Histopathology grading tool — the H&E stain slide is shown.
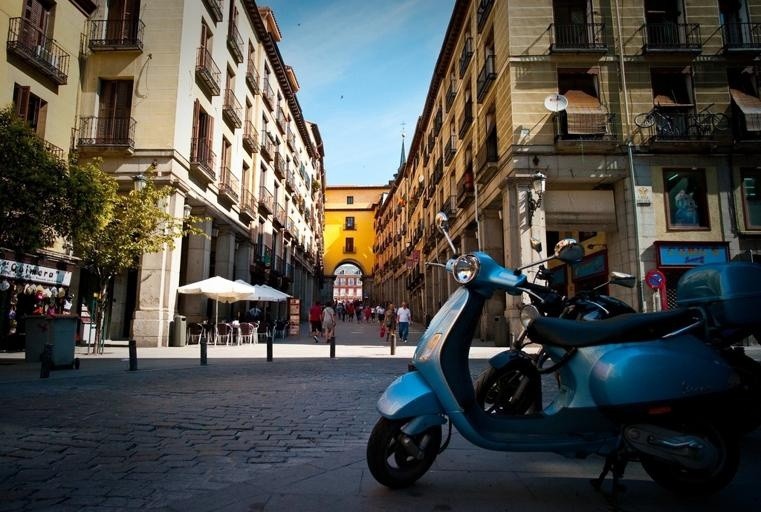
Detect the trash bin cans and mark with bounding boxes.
[24,314,82,371]
[172,315,187,347]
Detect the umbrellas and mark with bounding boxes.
[178,275,293,346]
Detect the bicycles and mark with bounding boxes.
[686,101,730,137]
[633,101,680,136]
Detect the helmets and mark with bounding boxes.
[473,351,542,418]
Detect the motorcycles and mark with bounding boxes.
[473,235,760,435]
[367,211,760,501]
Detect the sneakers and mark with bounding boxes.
[313,336,319,342]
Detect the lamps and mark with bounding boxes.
[526,171,548,227]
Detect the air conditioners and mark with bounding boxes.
[34,45,58,75]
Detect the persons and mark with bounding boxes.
[310,301,412,343]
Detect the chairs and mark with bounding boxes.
[186,320,291,347]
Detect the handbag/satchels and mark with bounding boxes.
[380,327,384,337]
[386,320,393,327]
